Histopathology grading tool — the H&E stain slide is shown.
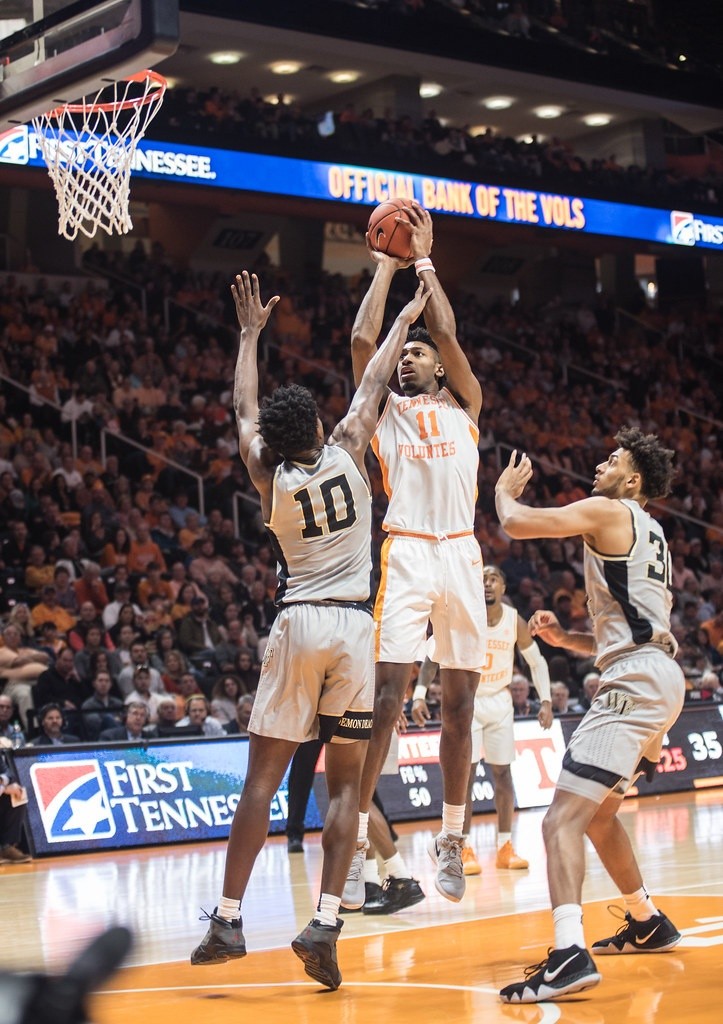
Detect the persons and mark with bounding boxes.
[0,242,723,863]
[412,566,554,875]
[350,0,696,74]
[342,203,487,909]
[191,271,435,991]
[27,86,723,216]
[494,427,686,1004]
[287,712,425,916]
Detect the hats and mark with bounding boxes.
[0,927,133,1024]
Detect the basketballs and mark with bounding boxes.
[368,198,428,260]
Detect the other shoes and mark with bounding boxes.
[1,842,24,861]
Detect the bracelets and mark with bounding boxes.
[415,257,436,276]
[413,685,428,701]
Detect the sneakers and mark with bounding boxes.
[292,918,343,990]
[363,879,383,903]
[287,836,303,853]
[461,848,480,874]
[341,838,370,910]
[191,907,246,967]
[592,904,681,954]
[363,876,425,915]
[500,950,603,1004]
[497,840,531,870]
[428,835,470,902]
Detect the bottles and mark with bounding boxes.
[11,720,22,750]
